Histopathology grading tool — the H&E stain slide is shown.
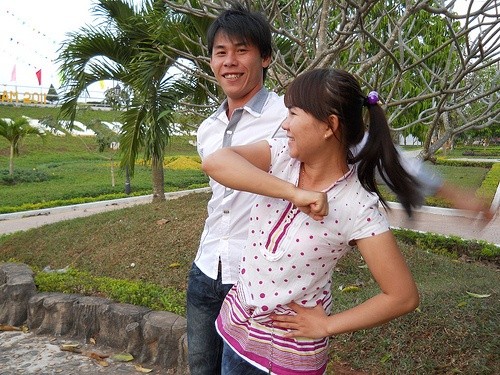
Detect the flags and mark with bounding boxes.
[99,69,105,89]
[10,65,17,82]
[36,70,41,85]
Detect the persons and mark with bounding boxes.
[198,67,428,375]
[186,2,497,375]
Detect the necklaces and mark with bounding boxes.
[302,163,305,190]
